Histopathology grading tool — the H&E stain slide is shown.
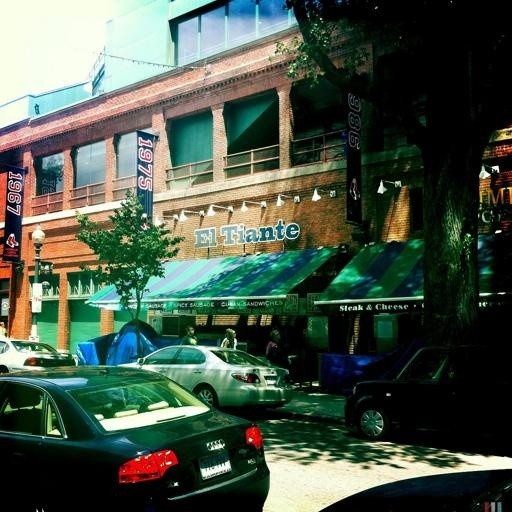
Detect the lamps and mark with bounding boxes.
[178,177,404,227]
[479,163,502,181]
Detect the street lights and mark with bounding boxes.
[28,225,47,343]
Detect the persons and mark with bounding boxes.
[178,325,198,346]
[220,328,238,362]
[0,322,8,336]
[266,328,281,367]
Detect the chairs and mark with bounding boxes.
[91,399,169,422]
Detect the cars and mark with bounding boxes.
[0,364,272,511]
[1,337,79,376]
[341,339,512,443]
[317,466,512,511]
[117,342,297,414]
